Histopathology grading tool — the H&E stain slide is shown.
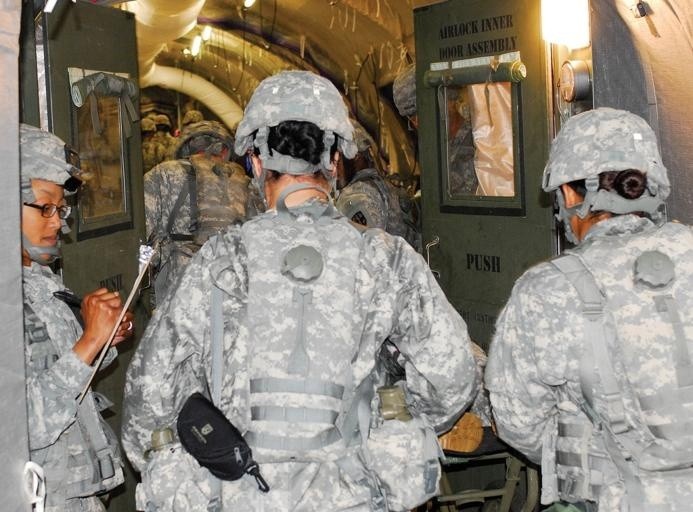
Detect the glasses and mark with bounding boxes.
[23,202,72,219]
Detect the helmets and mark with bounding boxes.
[174,121,234,160]
[541,107,672,200]
[182,110,203,125]
[140,112,172,132]
[393,62,458,117]
[234,69,379,159]
[19,123,83,198]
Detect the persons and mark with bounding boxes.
[19,124,138,509]
[121,70,478,510]
[78,90,422,310]
[481,108,693,511]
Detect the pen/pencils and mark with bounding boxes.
[52,292,81,309]
